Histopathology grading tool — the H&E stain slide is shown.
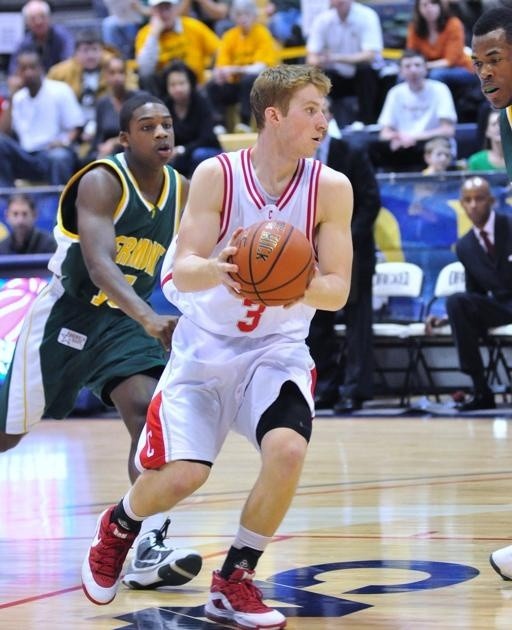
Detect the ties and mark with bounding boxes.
[481,230,495,255]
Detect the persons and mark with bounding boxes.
[470,8,512,586]
[78,64,357,628]
[2,95,203,590]
[308,2,512,411]
[1,1,300,273]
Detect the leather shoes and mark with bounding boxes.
[455,389,497,411]
[315,396,361,413]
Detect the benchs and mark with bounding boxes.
[0,1,512,258]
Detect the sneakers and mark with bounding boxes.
[120,528,201,590]
[81,506,137,604]
[204,569,286,630]
[489,542,512,581]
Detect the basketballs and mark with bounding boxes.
[226,220,316,306]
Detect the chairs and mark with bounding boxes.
[403,256,511,412]
[482,321,512,397]
[325,258,432,414]
